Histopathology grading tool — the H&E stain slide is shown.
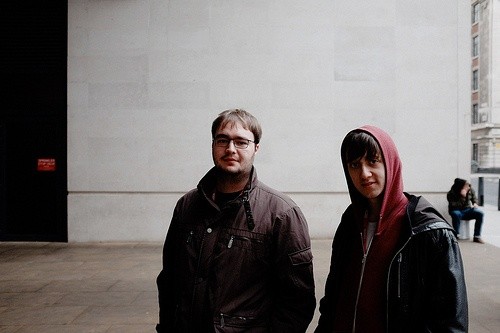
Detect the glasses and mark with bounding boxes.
[214,135,260,149]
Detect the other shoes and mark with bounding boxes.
[474,235,487,245]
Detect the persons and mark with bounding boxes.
[313,125,469,333]
[447,178,486,245]
[156,108,316,333]
